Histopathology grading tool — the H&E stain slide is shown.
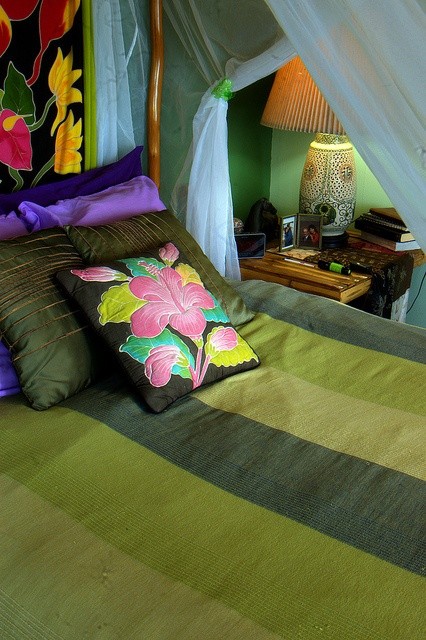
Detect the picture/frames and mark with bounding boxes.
[279,216,297,250]
[296,214,323,249]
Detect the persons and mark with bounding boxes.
[300,227,310,247]
[284,223,293,246]
[309,225,320,247]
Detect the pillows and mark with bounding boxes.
[17,175,163,227]
[0,226,113,410]
[52,242,261,414]
[63,209,256,328]
[0,212,32,397]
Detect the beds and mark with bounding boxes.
[0,160,425,636]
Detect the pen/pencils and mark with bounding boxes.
[282,258,315,268]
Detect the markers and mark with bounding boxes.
[317,259,352,277]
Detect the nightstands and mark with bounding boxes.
[240,204,424,305]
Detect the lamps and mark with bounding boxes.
[260,28,390,240]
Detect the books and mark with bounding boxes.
[350,207,422,256]
